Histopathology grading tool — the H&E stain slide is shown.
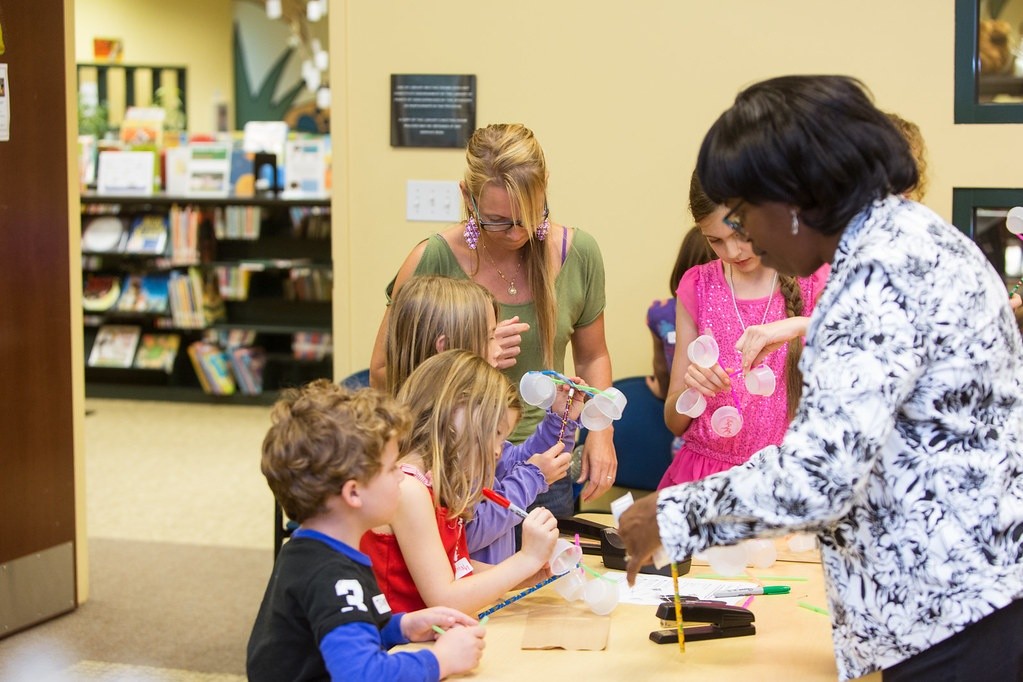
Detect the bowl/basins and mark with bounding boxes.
[687,335,719,369]
[693,530,818,576]
[711,406,743,438]
[745,365,776,397]
[675,388,707,418]
[580,399,613,431]
[549,538,621,618]
[593,387,628,420]
[518,370,557,409]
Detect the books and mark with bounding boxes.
[82,203,333,396]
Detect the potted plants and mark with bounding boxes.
[76,101,107,186]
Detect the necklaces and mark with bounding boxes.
[729,264,778,333]
[483,245,525,295]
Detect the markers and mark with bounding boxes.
[482,488,529,519]
[713,585,791,598]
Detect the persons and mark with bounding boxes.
[369,123,618,552]
[646,170,833,492]
[247,377,486,682]
[360,349,559,615]
[615,73,1023,682]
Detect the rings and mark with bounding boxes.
[607,476,613,480]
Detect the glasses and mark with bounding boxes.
[470,192,549,232]
[723,196,748,230]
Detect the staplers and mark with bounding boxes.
[649,603,756,644]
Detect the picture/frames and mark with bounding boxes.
[953,0,1023,124]
[951,186,1023,339]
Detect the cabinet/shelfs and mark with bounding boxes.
[80,195,332,405]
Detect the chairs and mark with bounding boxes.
[271,368,373,556]
[581,372,675,512]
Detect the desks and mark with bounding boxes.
[381,513,887,682]
